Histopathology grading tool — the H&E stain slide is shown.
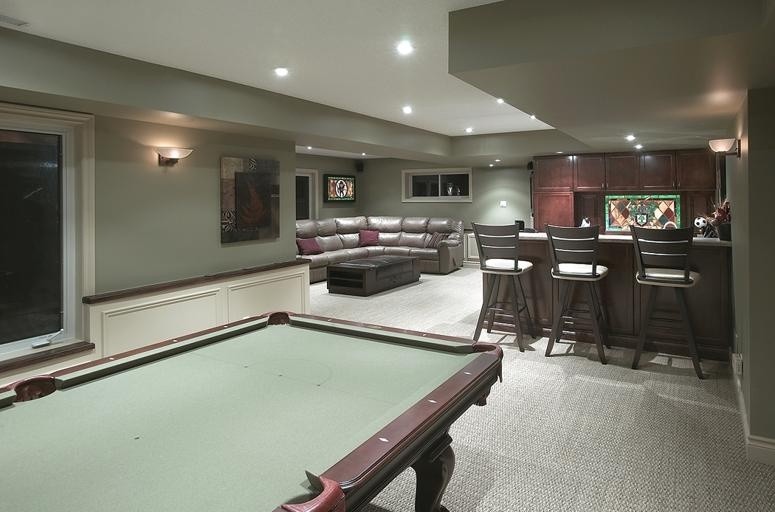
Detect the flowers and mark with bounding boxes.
[698,196,731,227]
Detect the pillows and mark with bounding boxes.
[296,239,323,255]
[359,229,379,247]
[426,232,449,248]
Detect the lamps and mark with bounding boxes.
[154,147,194,166]
[709,138,741,157]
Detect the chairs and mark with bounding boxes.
[545,223,610,364]
[471,221,536,352]
[630,225,702,379]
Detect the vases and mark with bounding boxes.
[719,224,731,240]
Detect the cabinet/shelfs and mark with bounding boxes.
[533,148,716,234]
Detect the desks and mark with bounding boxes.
[0,311,503,512]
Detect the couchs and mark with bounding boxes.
[296,216,464,283]
